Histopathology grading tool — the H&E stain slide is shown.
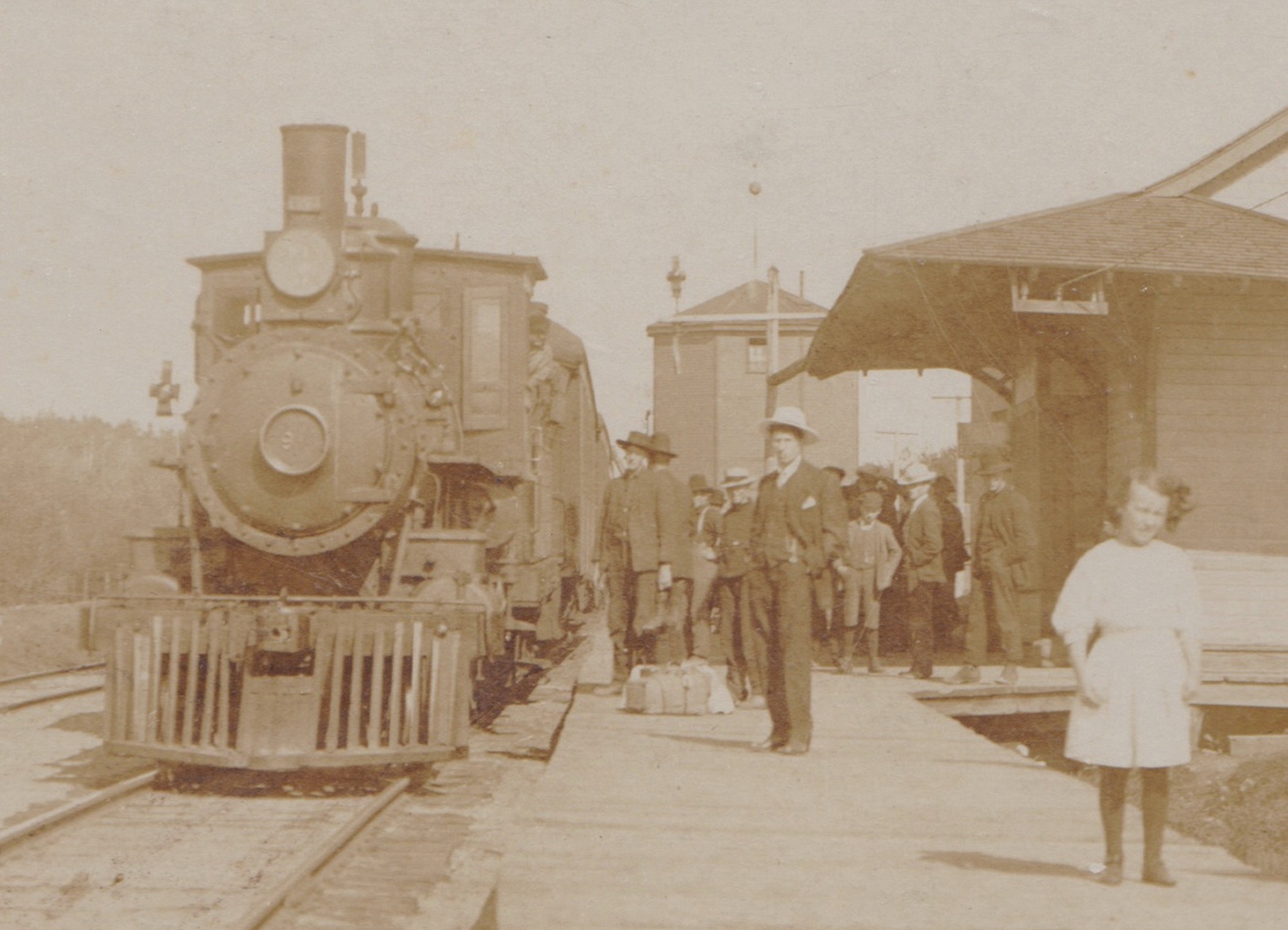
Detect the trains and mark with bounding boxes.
[74,123,613,784]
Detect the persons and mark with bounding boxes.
[589,406,1032,756]
[1048,463,1200,890]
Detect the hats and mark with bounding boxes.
[823,466,846,477]
[897,464,938,485]
[688,475,714,494]
[761,407,819,444]
[617,431,651,453]
[931,477,958,493]
[857,463,882,482]
[857,492,883,509]
[720,467,757,487]
[708,489,727,504]
[973,453,1013,476]
[638,432,679,457]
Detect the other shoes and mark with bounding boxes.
[1143,866,1177,886]
[836,657,1021,687]
[593,679,624,696]
[1107,864,1121,884]
[754,739,808,755]
[735,691,769,710]
